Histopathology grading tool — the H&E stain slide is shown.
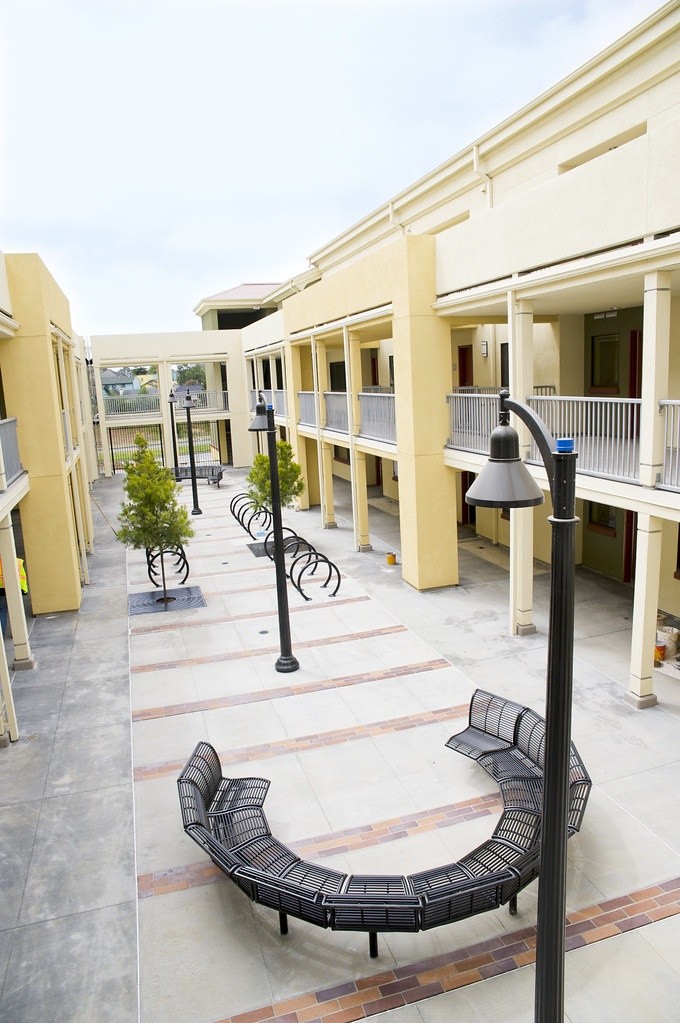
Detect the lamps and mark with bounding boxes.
[481,340,488,357]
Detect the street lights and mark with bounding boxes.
[167,388,182,482]
[463,387,579,1023]
[183,389,202,514]
[247,390,300,673]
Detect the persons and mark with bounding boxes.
[0,558,28,641]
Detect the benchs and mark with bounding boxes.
[168,465,227,489]
[176,689,592,960]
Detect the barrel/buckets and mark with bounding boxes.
[657,626,679,655]
[386,552,396,565]
[654,642,666,661]
